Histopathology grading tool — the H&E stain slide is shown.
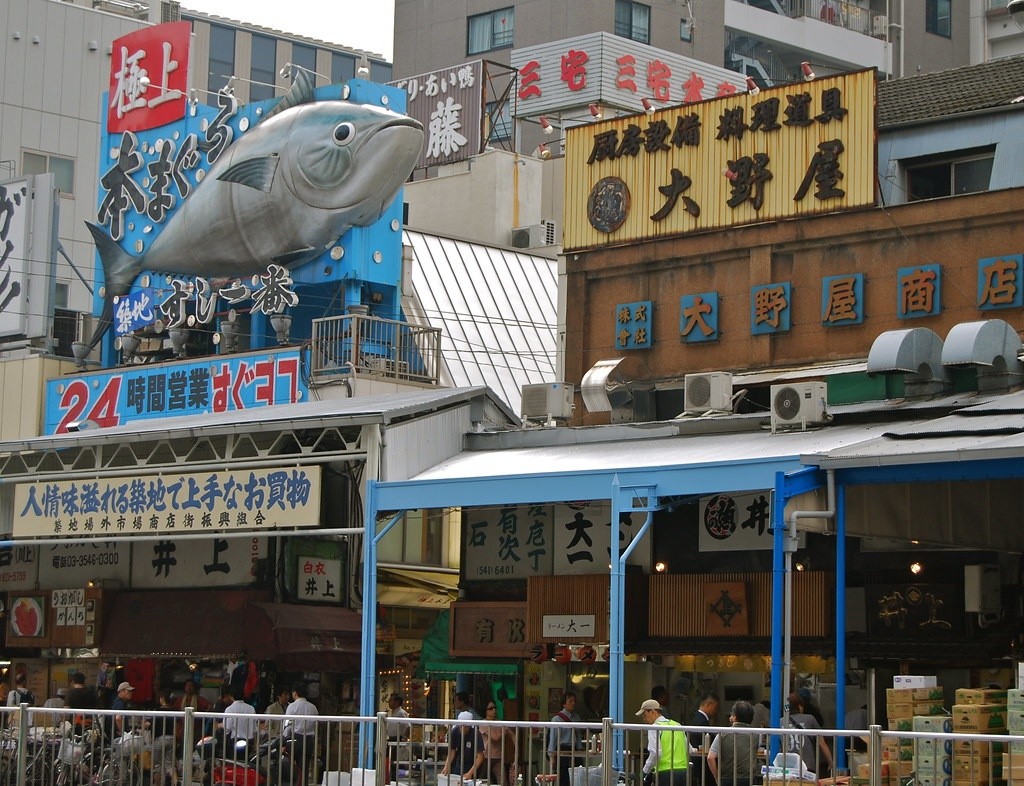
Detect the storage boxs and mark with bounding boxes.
[761,662,1024,786]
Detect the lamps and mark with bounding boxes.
[653,558,672,574]
[184,657,209,673]
[538,142,550,160]
[222,75,288,95]
[88,576,102,588]
[745,75,760,97]
[70,339,94,373]
[641,96,656,116]
[188,87,244,108]
[120,332,142,365]
[588,102,602,121]
[168,326,191,357]
[796,552,814,571]
[114,656,124,670]
[279,63,331,85]
[268,314,293,346]
[540,115,554,135]
[220,320,242,353]
[344,304,371,332]
[800,62,816,83]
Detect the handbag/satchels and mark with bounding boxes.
[119,730,133,744]
[398,747,409,761]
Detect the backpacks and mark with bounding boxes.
[16,689,34,707]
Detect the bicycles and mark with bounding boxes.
[0,716,303,786]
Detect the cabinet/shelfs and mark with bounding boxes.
[386,742,449,782]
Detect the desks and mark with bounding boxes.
[541,749,770,786]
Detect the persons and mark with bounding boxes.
[143,689,181,786]
[263,684,318,785]
[105,681,135,741]
[780,692,833,773]
[546,691,584,786]
[634,699,698,786]
[800,688,824,727]
[843,703,867,768]
[707,700,764,786]
[751,700,770,724]
[385,692,412,781]
[0,671,96,739]
[651,685,672,719]
[687,693,717,786]
[173,678,212,734]
[211,686,258,762]
[440,692,516,786]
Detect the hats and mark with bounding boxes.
[117,682,134,691]
[57,688,68,695]
[457,711,473,721]
[636,699,661,716]
[799,688,810,698]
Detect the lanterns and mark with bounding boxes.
[530,645,610,665]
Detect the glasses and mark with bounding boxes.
[485,707,497,711]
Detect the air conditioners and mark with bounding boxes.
[769,381,829,426]
[511,218,556,248]
[371,359,410,381]
[681,370,734,411]
[519,381,578,421]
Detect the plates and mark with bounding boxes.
[11,598,42,636]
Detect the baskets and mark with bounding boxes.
[568,766,619,786]
[113,734,143,756]
[57,739,87,765]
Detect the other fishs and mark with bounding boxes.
[86,70,426,350]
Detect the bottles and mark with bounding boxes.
[705,733,711,753]
[591,734,597,752]
